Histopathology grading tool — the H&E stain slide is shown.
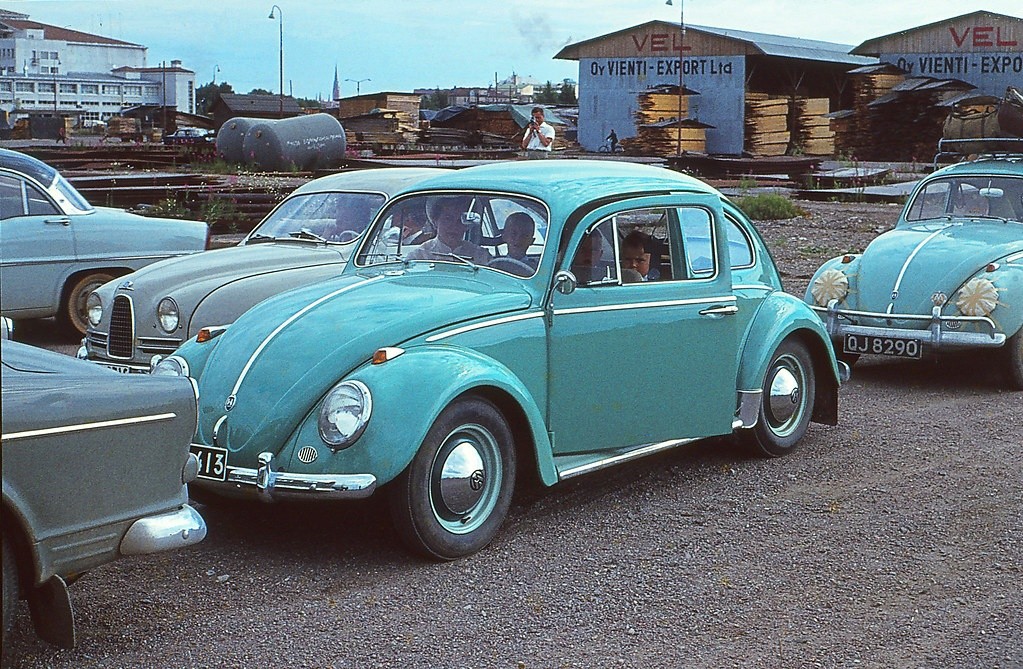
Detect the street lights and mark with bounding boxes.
[53,57,62,111]
[665,0,684,158]
[213,64,220,83]
[268,5,282,119]
[345,78,372,96]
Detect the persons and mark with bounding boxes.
[396,200,430,246]
[323,198,367,240]
[498,211,539,263]
[405,197,490,268]
[620,229,654,284]
[522,107,555,160]
[606,130,618,152]
[573,230,603,268]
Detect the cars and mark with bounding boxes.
[802,138,1023,391]
[2,145,209,342]
[74,168,545,374]
[0,315,209,650]
[164,128,211,144]
[147,158,842,562]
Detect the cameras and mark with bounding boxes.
[530,116,536,125]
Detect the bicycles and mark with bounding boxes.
[598,140,624,156]
